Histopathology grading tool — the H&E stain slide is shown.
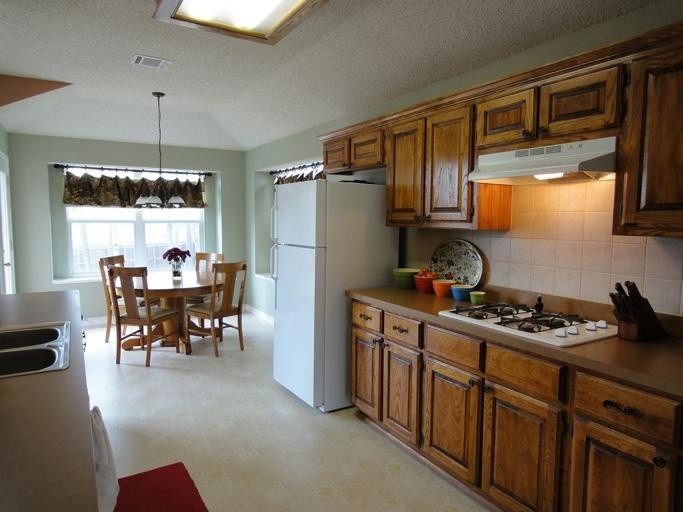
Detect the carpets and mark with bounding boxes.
[110,461,210,512]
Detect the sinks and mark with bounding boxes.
[1,348,70,379]
[0,321,70,350]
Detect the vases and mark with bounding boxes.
[169,258,183,281]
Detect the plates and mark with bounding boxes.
[430,239,483,288]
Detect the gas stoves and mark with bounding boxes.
[436,301,618,347]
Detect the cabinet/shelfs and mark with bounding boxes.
[471,61,626,154]
[561,365,682,512]
[381,103,512,234]
[320,126,386,175]
[422,322,568,511]
[346,300,422,454]
[609,41,682,240]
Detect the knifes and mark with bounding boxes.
[609,280,641,318]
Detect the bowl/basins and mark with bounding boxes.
[392,268,473,302]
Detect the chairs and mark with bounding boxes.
[182,260,249,356]
[182,250,226,338]
[104,261,179,369]
[97,254,151,343]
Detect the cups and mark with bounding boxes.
[469,291,486,304]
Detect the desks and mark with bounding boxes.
[104,269,225,348]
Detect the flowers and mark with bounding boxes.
[160,245,191,273]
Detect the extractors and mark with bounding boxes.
[468,136,616,186]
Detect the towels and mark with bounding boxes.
[89,403,122,512]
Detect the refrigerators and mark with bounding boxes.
[269,179,399,413]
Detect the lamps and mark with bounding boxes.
[150,0,327,50]
[132,91,186,212]
